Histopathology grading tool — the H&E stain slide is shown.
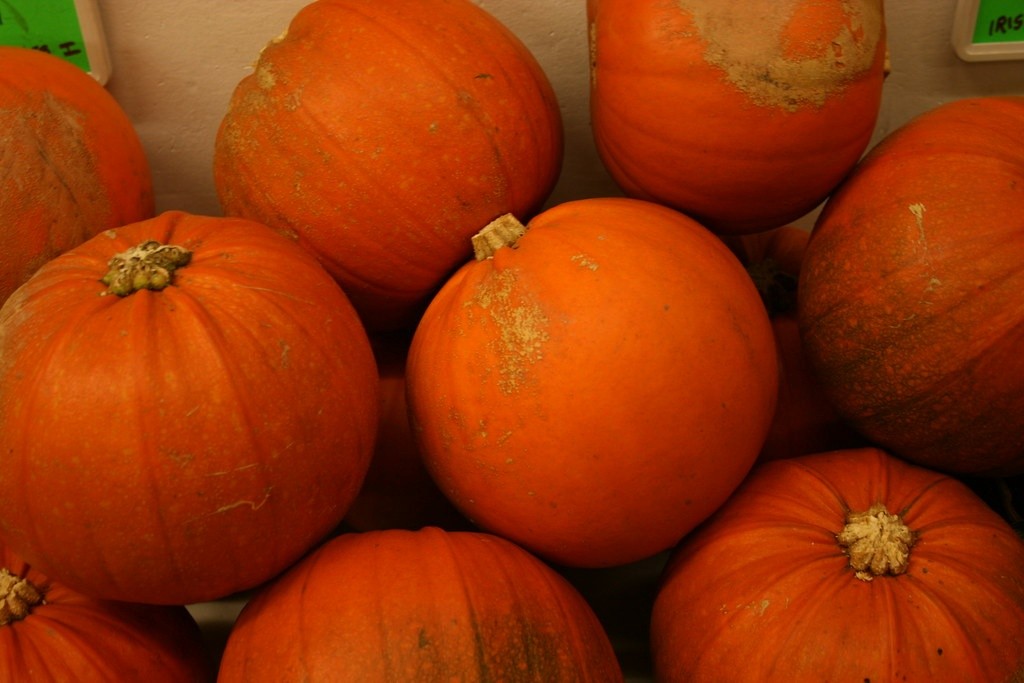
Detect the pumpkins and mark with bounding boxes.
[0,0,1024,683]
